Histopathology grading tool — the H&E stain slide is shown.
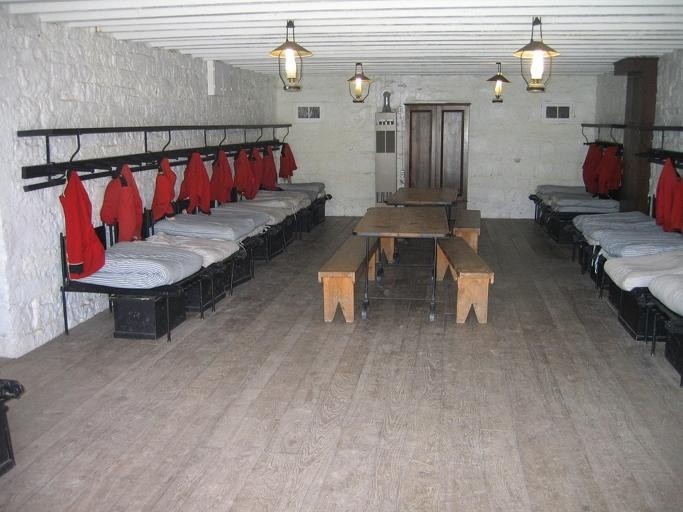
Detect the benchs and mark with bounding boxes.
[435,237,494,324]
[316,234,379,323]
[375,237,397,265]
[452,207,480,254]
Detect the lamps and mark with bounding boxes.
[483,62,511,103]
[346,63,373,103]
[266,19,314,91]
[510,16,563,94]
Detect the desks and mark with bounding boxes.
[351,207,450,322]
[384,187,460,208]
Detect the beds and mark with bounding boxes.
[237,181,311,233]
[105,207,240,314]
[143,202,263,290]
[56,219,206,343]
[597,245,683,356]
[206,190,287,261]
[569,194,683,273]
[647,271,683,391]
[260,177,331,223]
[226,186,301,251]
[177,198,271,277]
[528,182,619,243]
[581,229,683,295]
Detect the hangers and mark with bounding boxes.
[16,124,295,192]
[580,121,683,171]
